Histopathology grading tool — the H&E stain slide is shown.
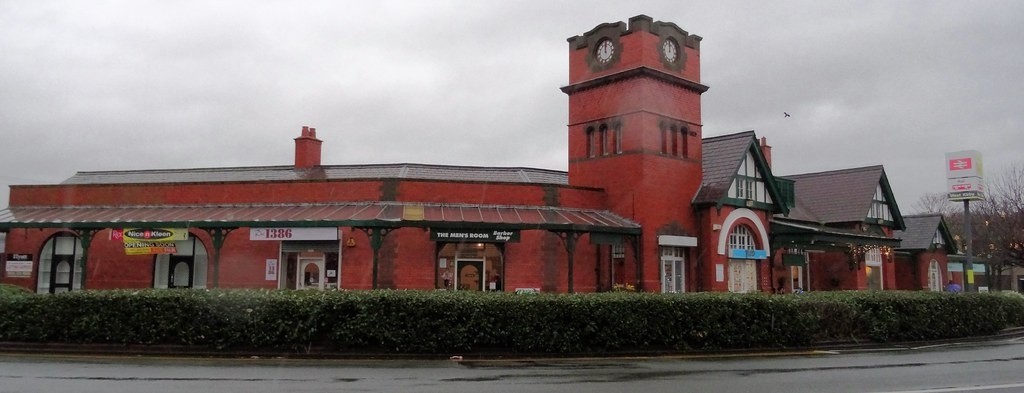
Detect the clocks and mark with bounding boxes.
[663,40,678,62]
[596,39,614,64]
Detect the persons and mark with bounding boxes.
[943,280,962,293]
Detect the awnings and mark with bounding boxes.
[770,218,902,248]
